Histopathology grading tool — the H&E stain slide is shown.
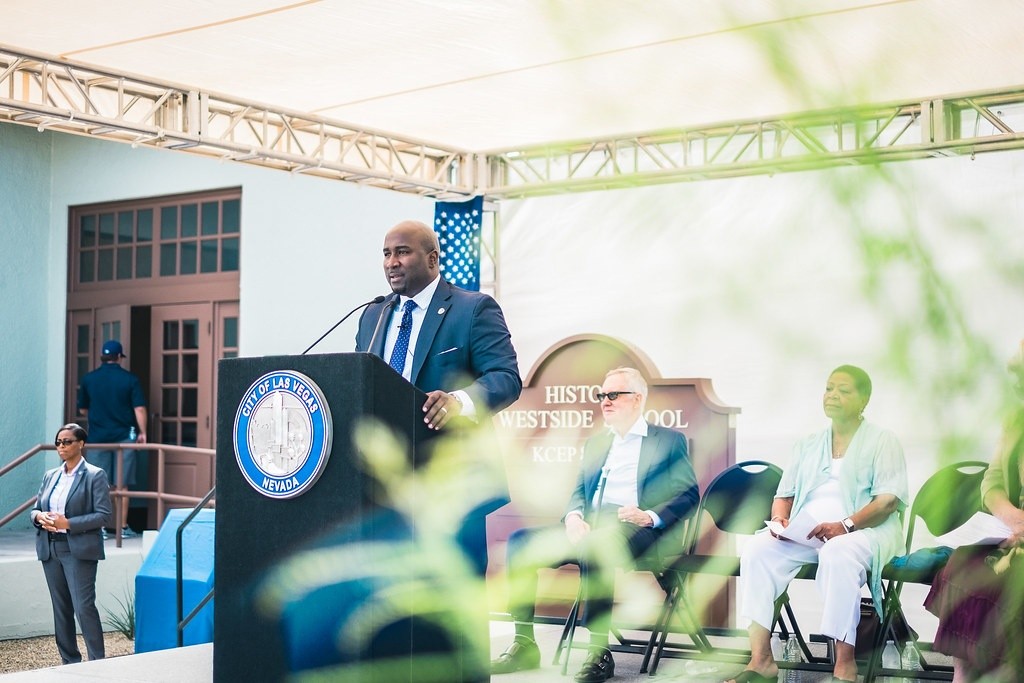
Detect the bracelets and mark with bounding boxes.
[840,520,849,533]
[771,516,778,521]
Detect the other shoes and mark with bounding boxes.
[574,647,616,682]
[492,640,541,675]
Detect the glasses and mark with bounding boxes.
[55,439,82,447]
[598,392,638,404]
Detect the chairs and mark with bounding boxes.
[865,461,989,683]
[756,548,915,681]
[648,460,815,678]
[552,485,703,665]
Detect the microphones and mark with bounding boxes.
[301,296,386,354]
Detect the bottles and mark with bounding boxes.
[768,632,785,683]
[900,641,921,683]
[882,640,901,683]
[128,426,137,440]
[783,634,803,683]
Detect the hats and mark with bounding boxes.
[102,339,126,358]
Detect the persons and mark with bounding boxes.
[922,341,1024,683]
[723,364,909,683]
[490,367,701,683]
[78,341,148,540]
[31,424,112,665]
[355,219,523,683]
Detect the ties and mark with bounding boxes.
[389,300,418,376]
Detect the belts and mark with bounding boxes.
[48,532,68,542]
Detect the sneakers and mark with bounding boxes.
[101,525,108,539]
[120,523,137,539]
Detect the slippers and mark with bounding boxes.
[830,674,858,683]
[722,669,779,683]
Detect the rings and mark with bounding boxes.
[823,536,827,542]
[441,407,447,413]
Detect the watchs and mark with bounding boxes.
[844,518,855,533]
